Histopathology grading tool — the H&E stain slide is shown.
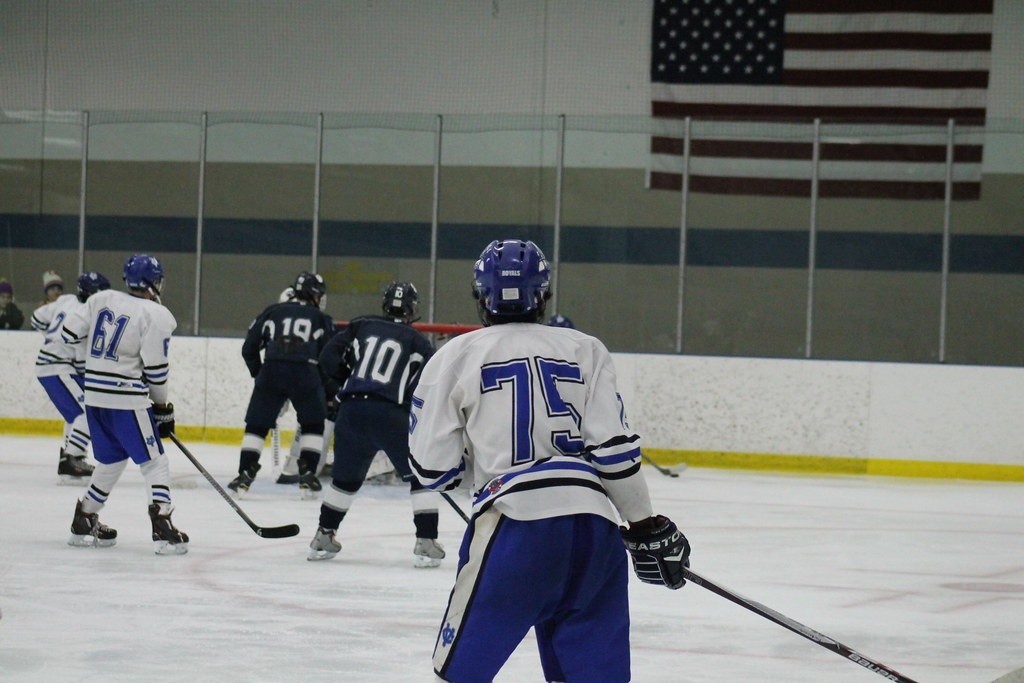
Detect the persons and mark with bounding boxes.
[37,271,64,308]
[0,282,25,330]
[309,280,446,560]
[30,271,112,477]
[62,254,190,544]
[226,271,337,493]
[408,240,691,683]
[547,314,576,329]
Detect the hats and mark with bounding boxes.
[42,271,63,293]
[0,280,12,293]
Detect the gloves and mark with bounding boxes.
[618,515,690,590]
[149,403,176,439]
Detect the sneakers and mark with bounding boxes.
[70,498,117,546]
[147,503,190,555]
[308,528,343,561]
[296,458,322,500]
[57,446,95,485]
[228,463,261,499]
[414,537,447,567]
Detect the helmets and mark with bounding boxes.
[123,254,165,298]
[548,315,574,328]
[294,271,326,300]
[77,272,110,298]
[382,281,418,317]
[471,239,551,322]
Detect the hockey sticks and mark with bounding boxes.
[641,454,688,477]
[441,491,471,524]
[619,526,1024,682]
[270,419,302,485]
[170,434,301,540]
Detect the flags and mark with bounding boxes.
[650,0,994,201]
